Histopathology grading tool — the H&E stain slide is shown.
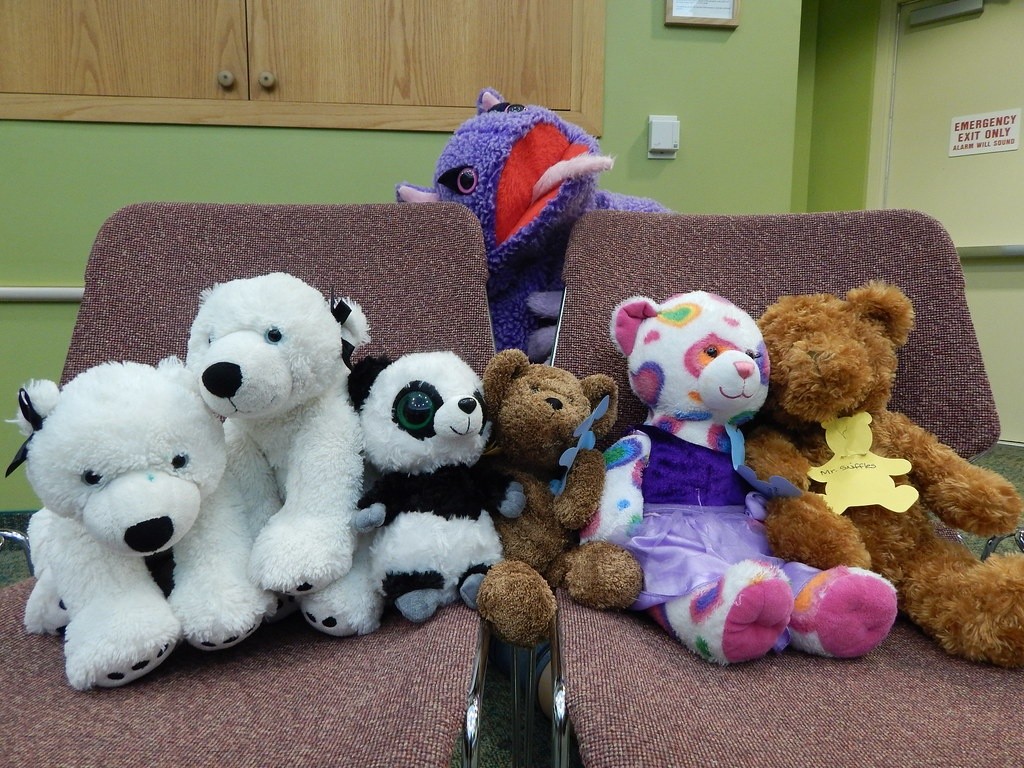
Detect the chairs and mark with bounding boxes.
[1,200,521,768]
[526,208,1024,768]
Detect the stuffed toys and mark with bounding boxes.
[347,350,524,621]
[397,89,674,362]
[477,349,643,644]
[184,272,385,636]
[580,291,897,664]
[745,281,1024,668]
[5,357,277,690]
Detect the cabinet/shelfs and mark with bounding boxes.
[0,0,605,140]
